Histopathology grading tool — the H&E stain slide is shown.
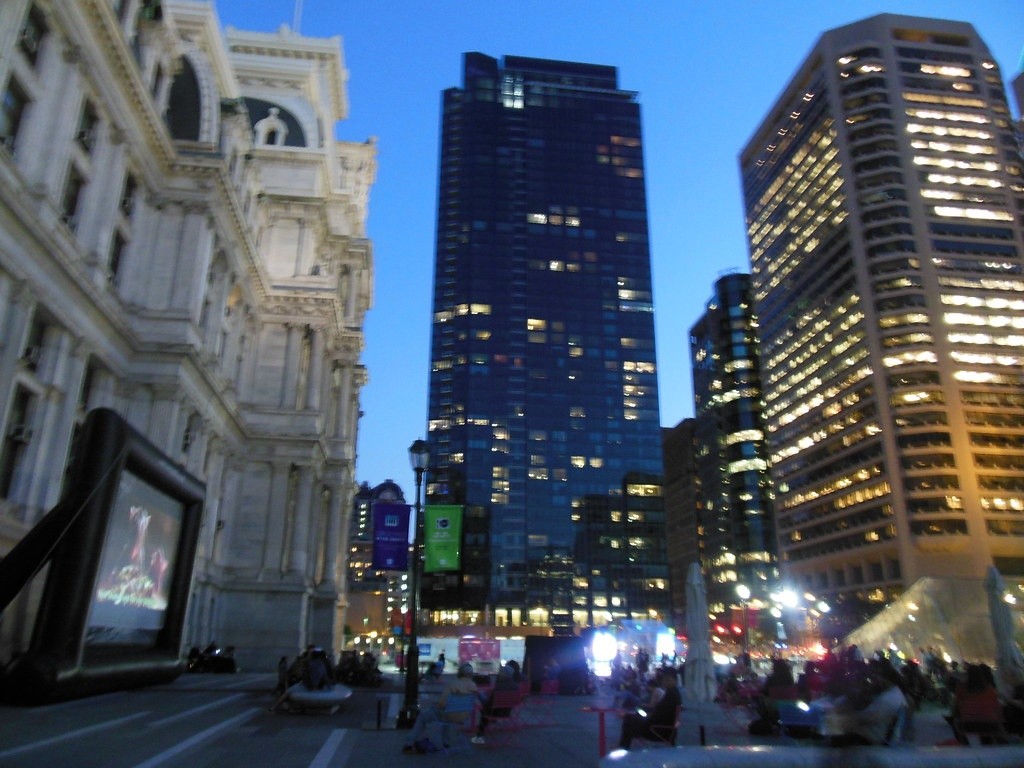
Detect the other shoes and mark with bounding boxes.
[401,745,413,754]
[263,708,277,716]
[471,737,485,745]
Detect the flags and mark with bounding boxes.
[389,607,402,634]
[422,504,464,572]
[405,607,415,635]
[370,501,416,573]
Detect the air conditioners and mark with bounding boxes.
[23,345,44,367]
[1,135,17,153]
[20,27,38,55]
[79,129,96,150]
[121,197,133,214]
[62,216,76,233]
[8,424,32,444]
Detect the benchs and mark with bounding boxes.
[288,681,352,715]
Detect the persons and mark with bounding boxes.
[604,648,697,748]
[396,652,524,756]
[277,642,382,691]
[714,631,1024,745]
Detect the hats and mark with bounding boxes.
[656,668,676,676]
[499,665,514,677]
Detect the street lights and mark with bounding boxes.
[396,440,430,731]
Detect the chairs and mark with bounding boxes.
[613,705,682,749]
[524,680,560,729]
[480,692,523,750]
[779,702,829,749]
[413,693,476,757]
[864,704,907,746]
